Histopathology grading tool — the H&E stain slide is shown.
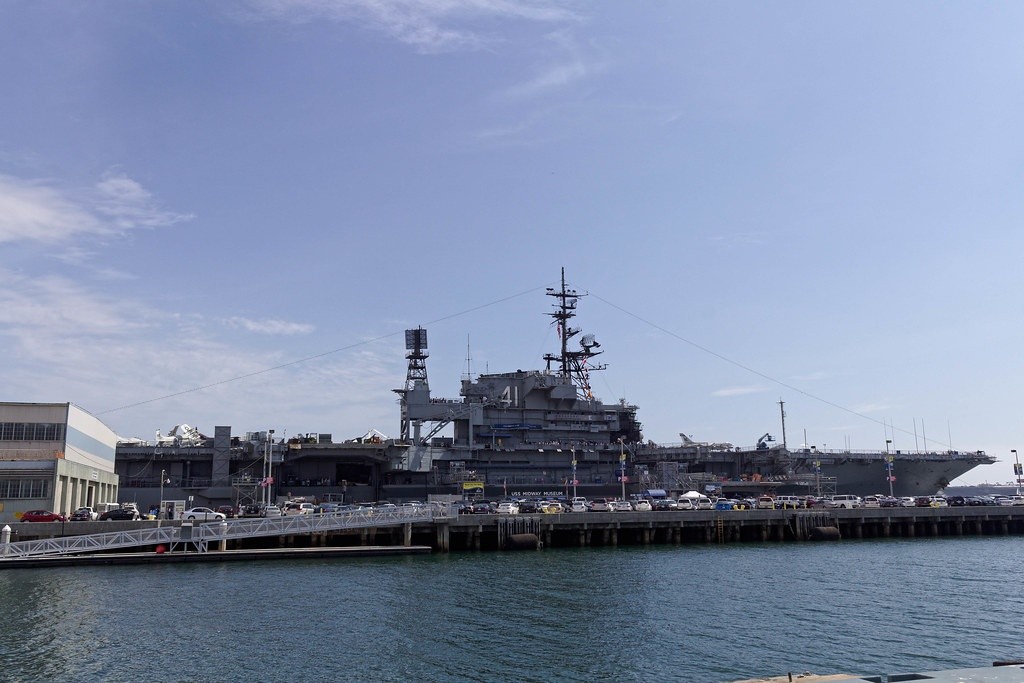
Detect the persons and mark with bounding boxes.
[147,504,160,520]
[469,468,475,480]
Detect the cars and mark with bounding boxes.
[650,496,775,510]
[20,509,68,522]
[70,502,139,521]
[216,500,438,518]
[177,506,226,520]
[450,496,653,514]
[863,493,1024,508]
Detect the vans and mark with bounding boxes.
[832,494,862,509]
[776,495,800,508]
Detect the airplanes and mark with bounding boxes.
[679,432,734,452]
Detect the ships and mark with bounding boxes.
[116,267,1002,495]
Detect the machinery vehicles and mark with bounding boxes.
[755,433,776,450]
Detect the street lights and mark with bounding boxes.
[811,445,821,497]
[1011,450,1022,496]
[570,446,576,497]
[617,436,625,501]
[161,470,170,500]
[885,439,894,497]
